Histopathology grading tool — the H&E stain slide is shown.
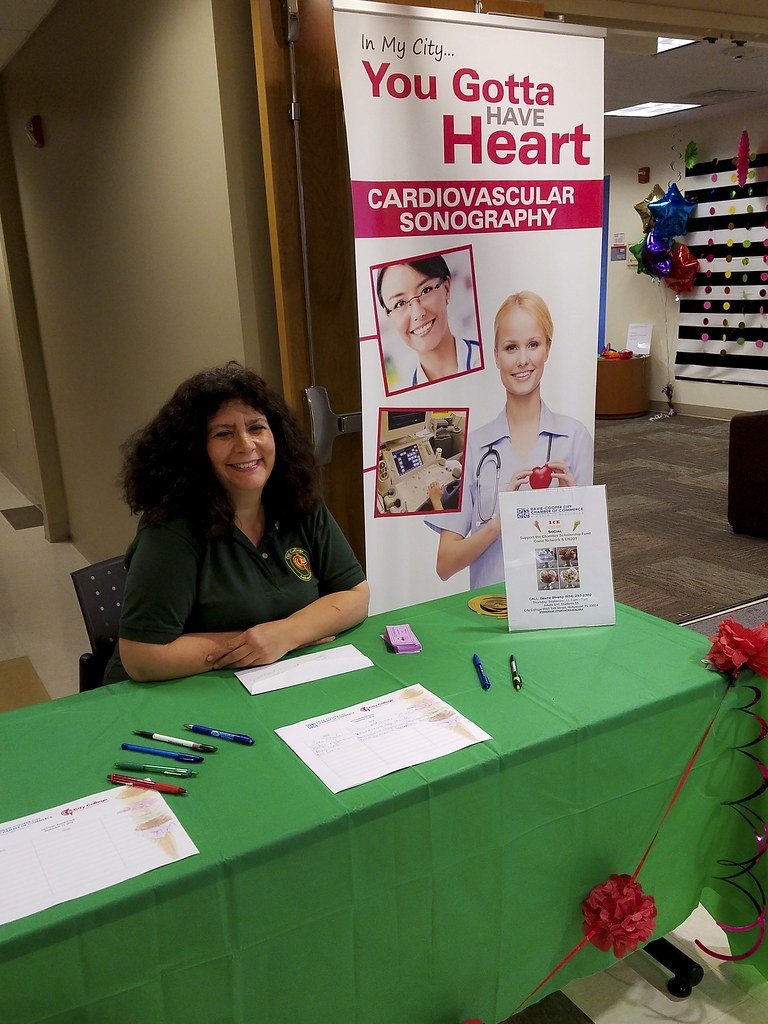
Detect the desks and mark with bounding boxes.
[0,587,768,1024]
[596,354,652,420]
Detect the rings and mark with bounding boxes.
[431,484,433,487]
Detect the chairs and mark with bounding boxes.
[68,548,132,692]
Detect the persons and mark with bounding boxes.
[378,255,482,384]
[425,290,593,589]
[101,360,371,687]
[428,482,444,510]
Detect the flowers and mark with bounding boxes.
[708,616,768,679]
[581,874,657,960]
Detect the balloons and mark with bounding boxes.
[629,182,699,294]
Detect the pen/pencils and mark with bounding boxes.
[106,773,188,795]
[113,760,199,778]
[131,729,219,753]
[473,653,491,690]
[121,743,205,764]
[510,653,523,691]
[183,723,254,744]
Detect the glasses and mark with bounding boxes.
[384,276,446,320]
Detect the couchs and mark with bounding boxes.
[727,410,768,540]
